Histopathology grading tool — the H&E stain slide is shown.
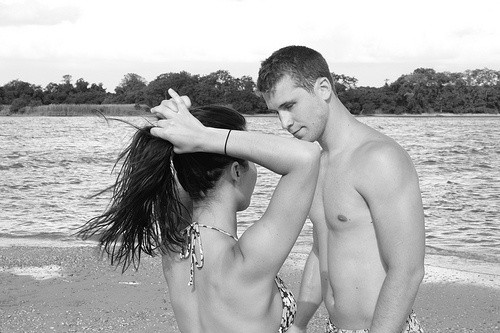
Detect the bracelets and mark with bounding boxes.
[224,129,231,157]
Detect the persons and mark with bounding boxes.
[129,87,320,333]
[257,46,425,333]
